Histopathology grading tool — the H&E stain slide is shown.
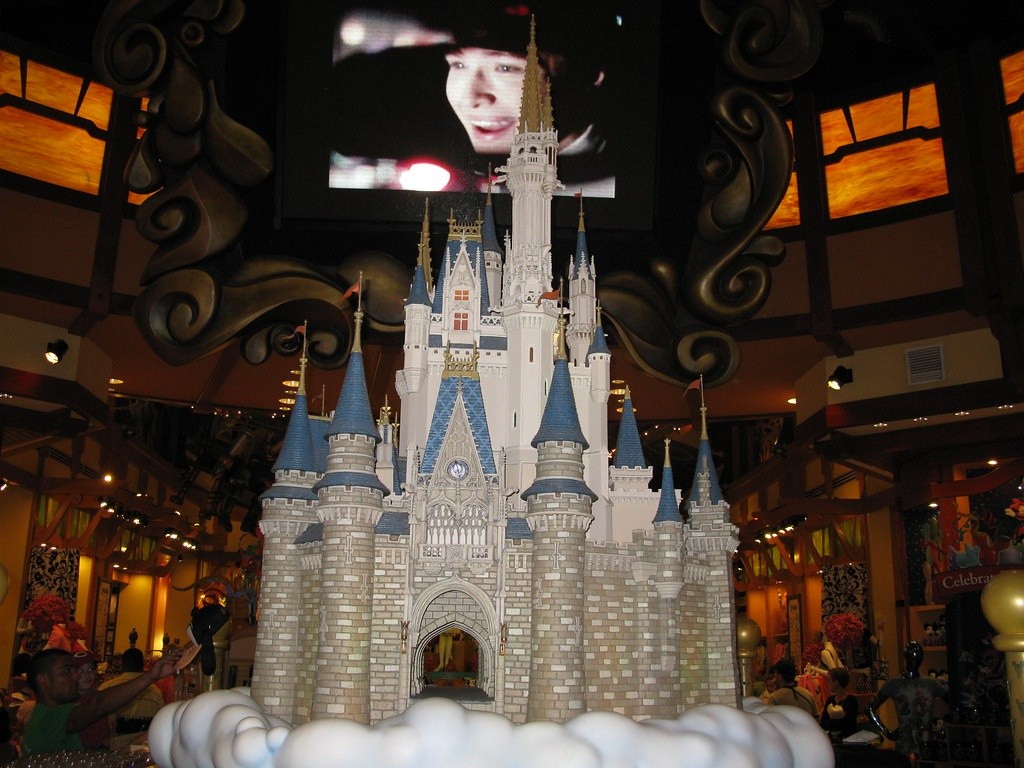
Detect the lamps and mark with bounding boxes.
[44,341,69,365]
[827,366,854,391]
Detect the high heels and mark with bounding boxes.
[433,665,444,673]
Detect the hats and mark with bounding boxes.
[73,650,96,665]
[190,606,230,676]
[12,654,32,676]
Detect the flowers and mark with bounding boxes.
[801,612,865,666]
[24,594,88,640]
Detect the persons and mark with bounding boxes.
[920,621,946,646]
[21,647,186,757]
[927,667,948,686]
[933,714,948,731]
[441,39,614,197]
[97,647,166,748]
[864,638,948,767]
[765,676,776,695]
[818,668,859,768]
[766,658,822,726]
[69,649,113,750]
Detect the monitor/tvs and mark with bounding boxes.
[280,0,659,231]
